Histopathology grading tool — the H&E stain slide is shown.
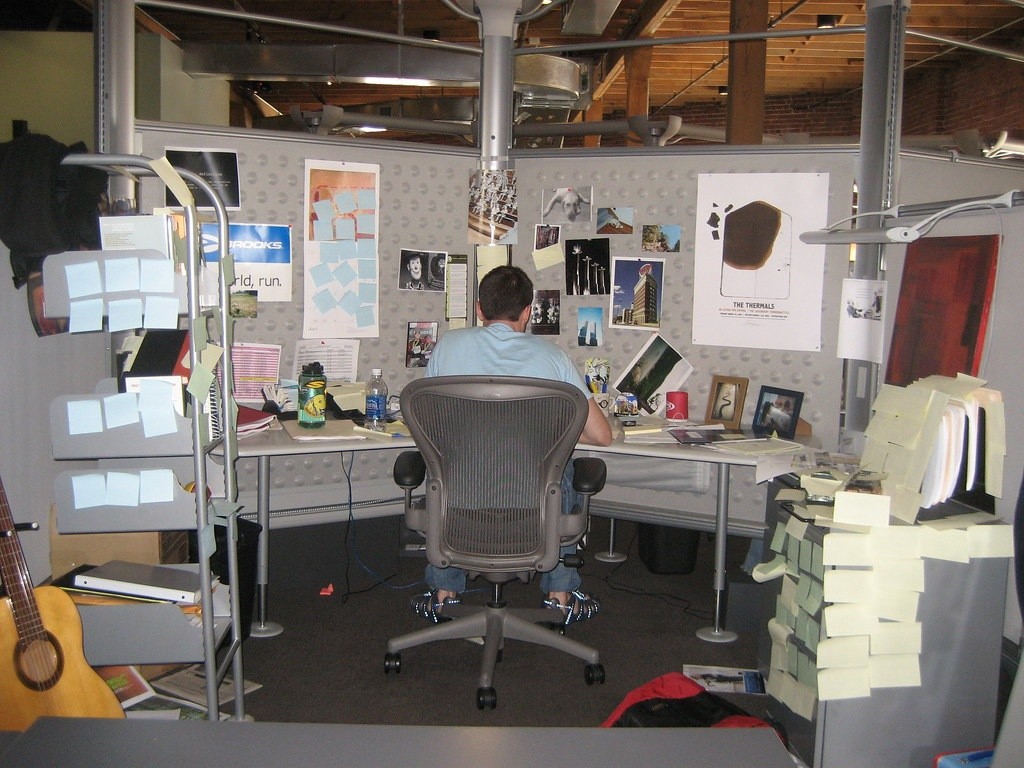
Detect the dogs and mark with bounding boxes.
[543,188,591,221]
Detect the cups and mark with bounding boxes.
[666,392,688,419]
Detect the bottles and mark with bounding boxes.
[364,368,388,433]
[296,361,328,429]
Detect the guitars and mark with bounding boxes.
[0,476,127,733]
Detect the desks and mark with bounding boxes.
[209,419,822,641]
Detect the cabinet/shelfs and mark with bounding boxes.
[0,147,259,724]
[757,469,1012,767]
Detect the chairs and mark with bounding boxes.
[384,374,608,709]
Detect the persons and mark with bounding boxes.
[761,397,800,432]
[410,331,422,358]
[406,254,425,290]
[413,265,599,624]
[420,334,437,368]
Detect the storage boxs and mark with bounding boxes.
[48,502,187,580]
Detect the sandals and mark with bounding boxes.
[543,589,599,625]
[410,588,461,626]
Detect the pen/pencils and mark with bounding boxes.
[354,425,402,440]
[585,375,607,393]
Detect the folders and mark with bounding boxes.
[74,559,220,603]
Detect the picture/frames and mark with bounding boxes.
[752,385,805,439]
[704,375,750,430]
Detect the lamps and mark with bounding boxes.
[798,187,1017,245]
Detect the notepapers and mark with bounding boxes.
[622,425,663,436]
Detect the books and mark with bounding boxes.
[671,424,770,450]
[50,559,265,723]
[231,398,412,442]
